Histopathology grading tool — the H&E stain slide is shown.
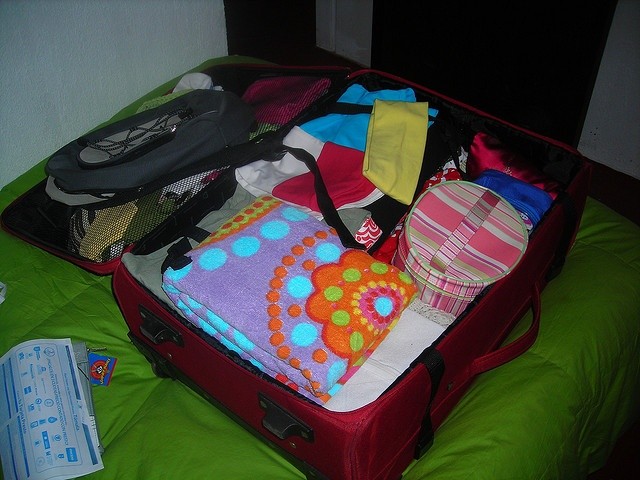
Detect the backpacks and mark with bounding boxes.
[35,88,254,209]
